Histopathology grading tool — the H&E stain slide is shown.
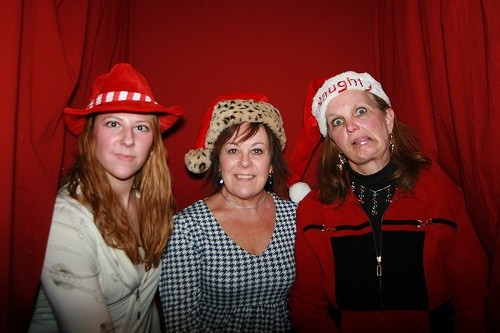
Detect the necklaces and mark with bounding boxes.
[220,189,266,210]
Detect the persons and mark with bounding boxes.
[28,62,183,333]
[286,70,489,333]
[158,92,297,333]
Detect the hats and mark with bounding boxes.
[182,92,287,175]
[65,63,187,137]
[286,70,393,206]
